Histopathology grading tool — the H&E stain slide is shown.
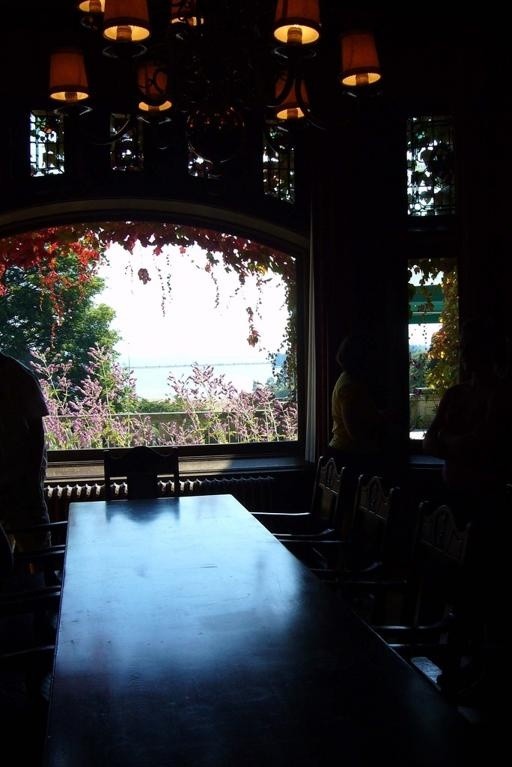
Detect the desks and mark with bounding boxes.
[44,494,505,766]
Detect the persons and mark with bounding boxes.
[329,338,366,450]
[423,383,477,452]
[1,355,50,557]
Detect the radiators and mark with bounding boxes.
[42,477,275,521]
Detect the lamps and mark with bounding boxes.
[40,0,383,153]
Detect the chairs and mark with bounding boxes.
[103,444,182,504]
[252,459,510,730]
[0,514,68,765]
